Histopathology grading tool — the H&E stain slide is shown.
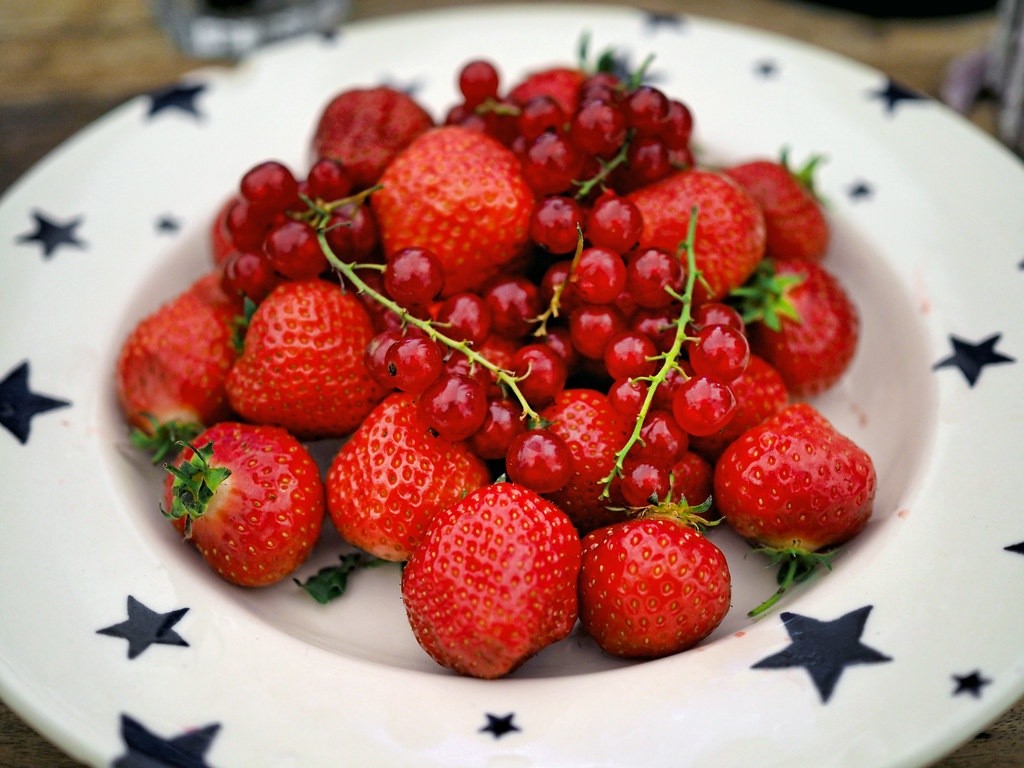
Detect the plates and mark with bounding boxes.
[0,0,1023,767]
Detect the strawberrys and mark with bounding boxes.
[115,69,879,679]
